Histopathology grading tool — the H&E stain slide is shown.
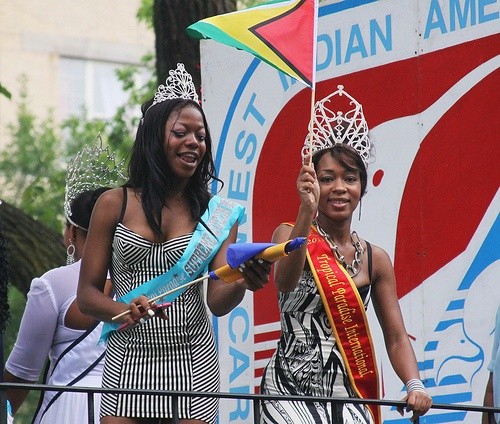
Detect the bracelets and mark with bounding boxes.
[405,378,426,394]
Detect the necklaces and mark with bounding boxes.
[312,218,365,279]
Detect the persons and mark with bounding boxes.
[0,132,132,424]
[77,62,271,424]
[259,85,432,424]
